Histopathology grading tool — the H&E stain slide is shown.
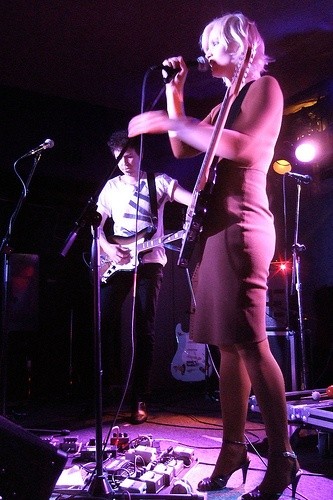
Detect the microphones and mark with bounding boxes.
[149,56,209,75]
[285,171,312,184]
[21,139,54,158]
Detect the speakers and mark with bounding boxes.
[264,263,288,331]
[0,415,68,500]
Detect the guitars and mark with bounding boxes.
[177,43,253,269]
[99,226,183,285]
[171,262,211,382]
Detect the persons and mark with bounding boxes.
[91,129,193,423]
[129,14,306,500]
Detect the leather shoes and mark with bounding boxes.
[132,400,148,424]
[80,399,104,417]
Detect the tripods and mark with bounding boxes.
[0,83,208,500]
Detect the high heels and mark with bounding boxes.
[198,439,251,491]
[241,451,303,500]
